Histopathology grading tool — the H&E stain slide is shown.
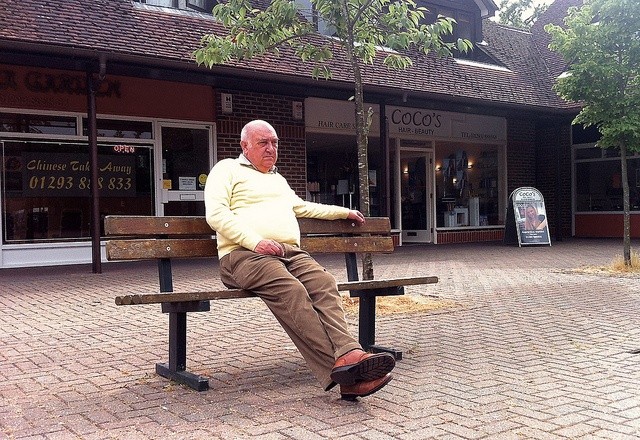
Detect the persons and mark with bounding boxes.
[204,119,396,400]
[524,204,541,231]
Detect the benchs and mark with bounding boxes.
[102,215,439,391]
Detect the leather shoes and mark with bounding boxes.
[329,348,396,386]
[339,372,393,401]
